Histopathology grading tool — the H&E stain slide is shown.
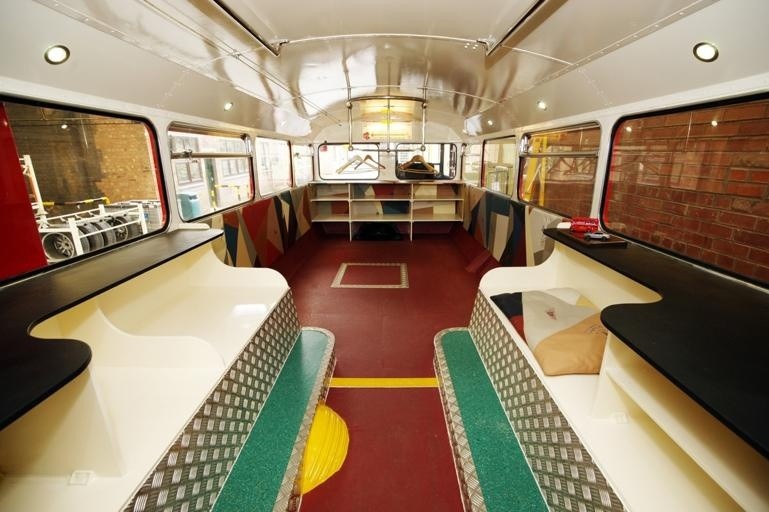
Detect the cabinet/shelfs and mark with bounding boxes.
[308,180,466,242]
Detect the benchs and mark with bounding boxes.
[0,225,301,511]
[469,221,769,511]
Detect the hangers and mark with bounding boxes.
[336,148,439,175]
